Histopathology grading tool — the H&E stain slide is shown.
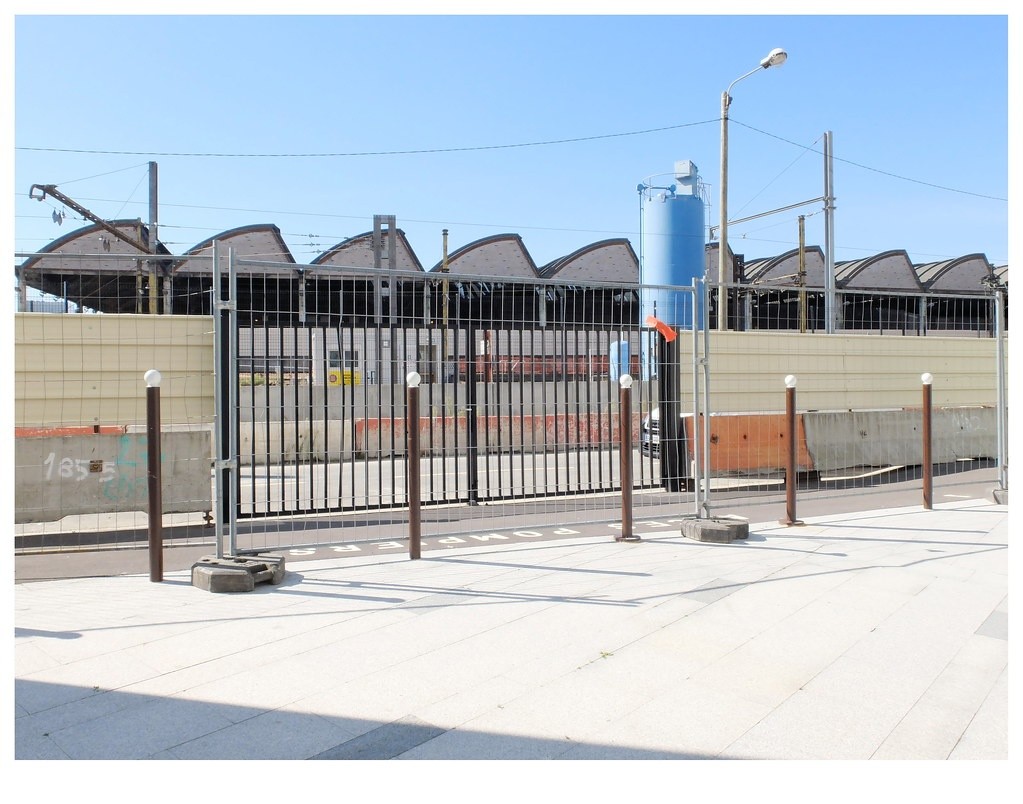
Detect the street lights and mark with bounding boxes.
[717,49,788,331]
